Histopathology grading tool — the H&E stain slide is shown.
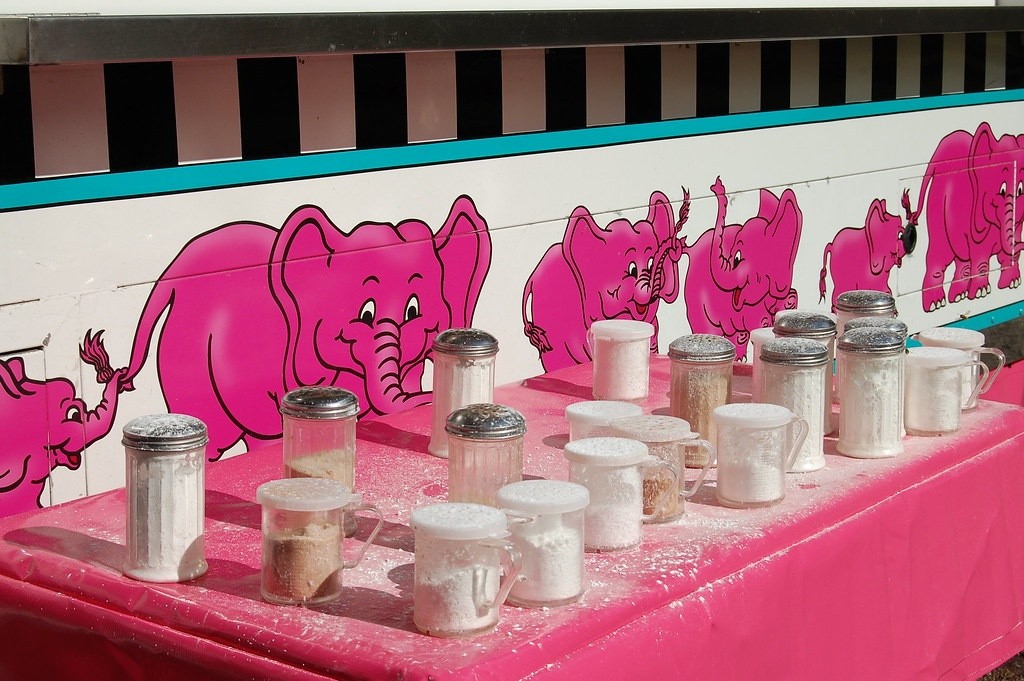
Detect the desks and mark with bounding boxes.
[0,356,1024,681]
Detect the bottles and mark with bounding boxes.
[122,413,208,583]
[667,333,736,468]
[430,328,499,459]
[844,316,906,438]
[445,402,526,504]
[773,312,836,435]
[279,386,362,492]
[834,289,898,405]
[838,329,907,459]
[759,337,829,473]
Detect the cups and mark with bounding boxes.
[615,416,716,526]
[714,402,810,510]
[919,328,1005,412]
[587,320,654,399]
[905,347,987,437]
[411,502,520,639]
[496,481,589,608]
[257,478,383,608]
[566,401,641,441]
[750,327,774,403]
[565,437,678,554]
